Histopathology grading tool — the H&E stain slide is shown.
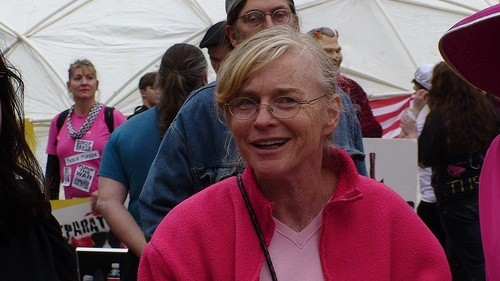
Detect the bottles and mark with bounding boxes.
[107,263,121,281]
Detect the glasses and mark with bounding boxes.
[225,94,330,120]
[236,9,294,29]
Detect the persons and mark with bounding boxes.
[98,1,500,281]
[0,51,79,281]
[43,58,131,281]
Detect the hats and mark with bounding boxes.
[438,3,500,100]
[199,21,226,48]
[413,63,436,91]
[225,0,294,19]
